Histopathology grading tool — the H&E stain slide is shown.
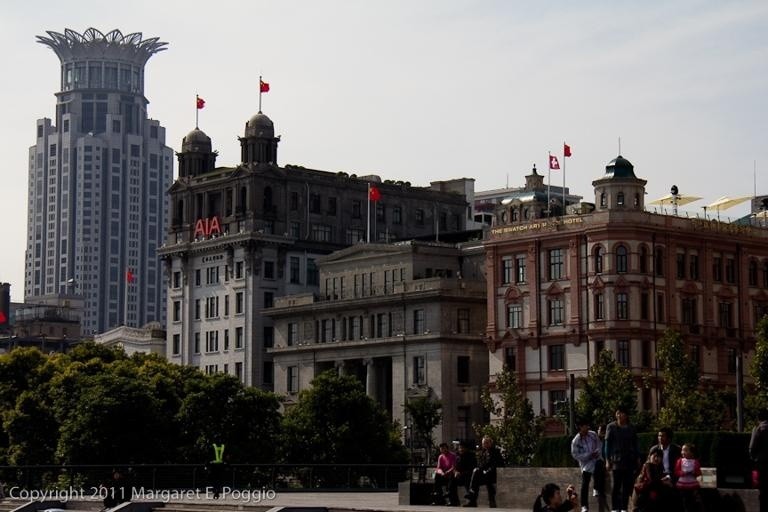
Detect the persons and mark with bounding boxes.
[418,404,764,512]
[204,433,230,499]
[102,470,132,511]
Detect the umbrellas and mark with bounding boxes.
[648,193,703,217]
[700,192,757,224]
[750,208,768,228]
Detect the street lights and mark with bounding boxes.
[553,398,568,436]
[402,423,414,478]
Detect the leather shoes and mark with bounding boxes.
[462,502,478,507]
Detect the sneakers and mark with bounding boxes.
[581,486,599,512]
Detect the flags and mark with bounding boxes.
[260,81,271,95]
[127,271,134,283]
[368,186,381,201]
[564,145,571,157]
[0,306,6,324]
[197,97,205,110]
[549,156,561,170]
